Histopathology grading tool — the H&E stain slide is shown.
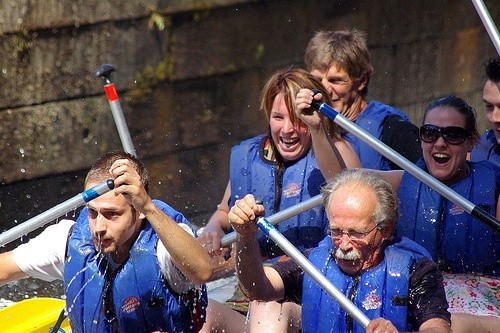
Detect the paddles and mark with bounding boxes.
[250,200,369,328]
[310,90,500,230]
[0,179,115,246]
[219,193,327,248]
[473,1,500,55]
[96,64,136,158]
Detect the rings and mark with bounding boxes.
[206,240,213,244]
[201,244,206,248]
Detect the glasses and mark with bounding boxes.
[324,219,386,240]
[418,124,471,145]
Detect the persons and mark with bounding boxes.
[466,55,500,165]
[304,25,423,171]
[194,63,364,333]
[227,168,452,333]
[379,94,500,333]
[0,150,214,333]
